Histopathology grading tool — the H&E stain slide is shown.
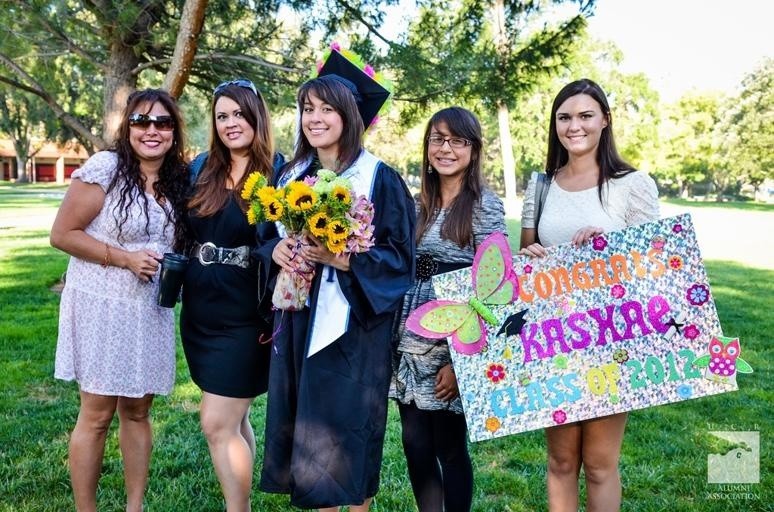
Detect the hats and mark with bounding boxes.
[315,48,391,133]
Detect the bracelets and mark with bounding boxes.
[103,243,111,268]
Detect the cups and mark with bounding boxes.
[146,251,190,309]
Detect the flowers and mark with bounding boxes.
[237,164,377,260]
[304,40,393,135]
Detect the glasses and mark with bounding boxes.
[427,133,476,148]
[211,78,259,97]
[128,113,178,130]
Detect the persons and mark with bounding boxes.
[516,78,660,512]
[386,107,508,512]
[50,89,177,512]
[252,74,416,511]
[173,79,288,512]
